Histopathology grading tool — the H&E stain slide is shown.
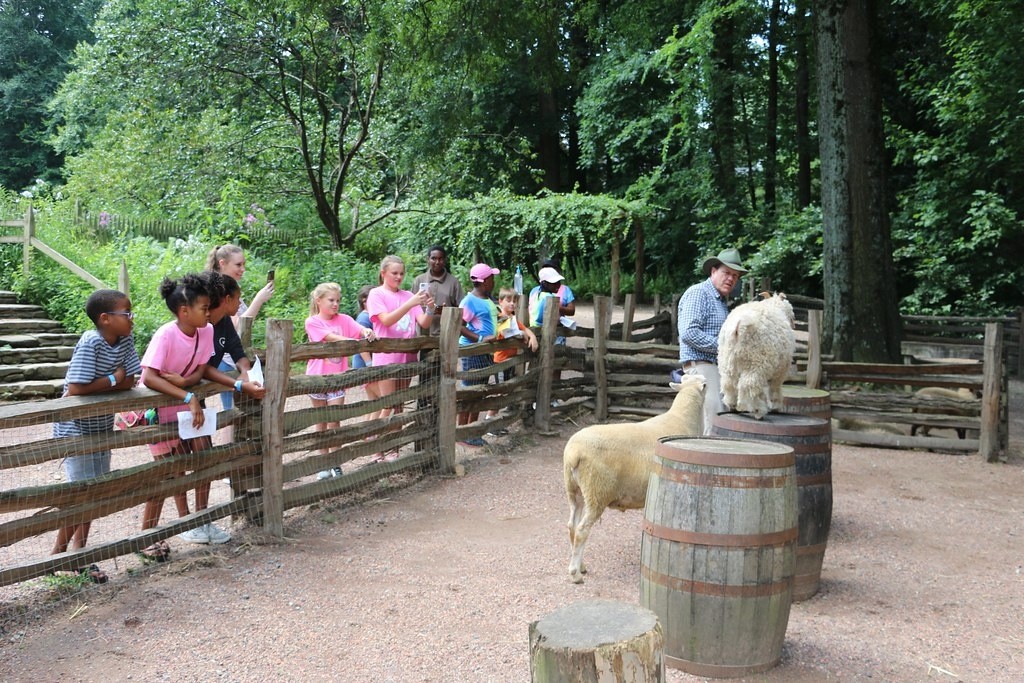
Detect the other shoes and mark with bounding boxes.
[317,470,333,479]
[456,438,488,448]
[487,429,509,436]
[333,467,343,477]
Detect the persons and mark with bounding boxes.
[352,255,435,463]
[677,248,749,438]
[305,282,380,481]
[174,274,264,544]
[137,274,216,561]
[529,260,575,408]
[54,290,141,583]
[411,246,467,337]
[485,287,538,437]
[459,263,498,448]
[205,244,275,485]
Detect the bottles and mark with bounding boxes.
[514,266,522,295]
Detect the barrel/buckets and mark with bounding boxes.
[778,385,831,420]
[640,435,798,676]
[709,411,834,602]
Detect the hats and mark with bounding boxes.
[703,249,749,278]
[538,267,565,283]
[470,263,500,282]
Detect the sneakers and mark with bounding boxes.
[177,528,209,543]
[200,523,231,544]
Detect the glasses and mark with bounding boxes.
[98,312,134,320]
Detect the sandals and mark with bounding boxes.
[79,563,108,583]
[52,569,77,577]
[137,546,167,562]
[159,541,169,552]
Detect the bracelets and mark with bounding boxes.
[365,361,372,366]
[236,380,243,391]
[183,391,193,404]
[426,306,434,316]
[478,334,483,341]
[108,374,116,387]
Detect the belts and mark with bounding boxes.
[684,360,711,369]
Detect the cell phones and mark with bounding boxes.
[267,270,275,283]
[420,283,428,292]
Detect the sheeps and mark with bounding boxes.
[563,374,708,583]
[716,291,795,421]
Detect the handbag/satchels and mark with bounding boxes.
[115,410,147,430]
